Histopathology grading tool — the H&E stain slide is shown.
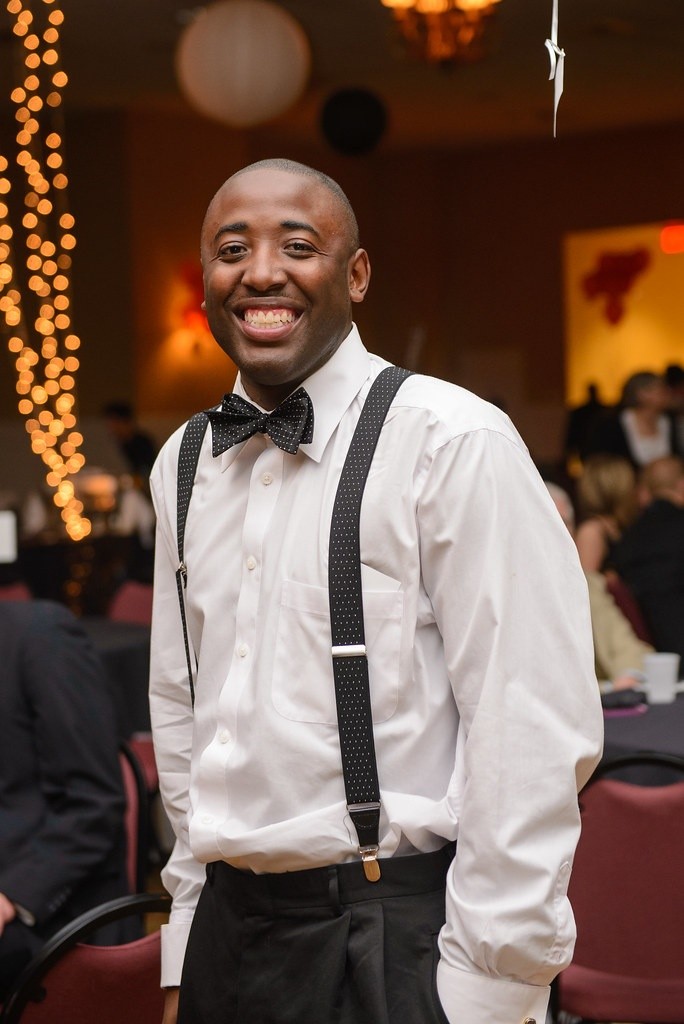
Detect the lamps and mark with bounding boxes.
[382,0,503,67]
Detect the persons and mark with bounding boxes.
[546,365,684,686]
[148,159,606,1023]
[0,599,119,1024]
[102,401,156,551]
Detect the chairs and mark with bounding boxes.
[118,735,150,945]
[550,752,684,1024]
[113,580,153,626]
[0,892,172,1024]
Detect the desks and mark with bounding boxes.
[80,616,151,734]
[599,690,684,785]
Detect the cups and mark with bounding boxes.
[643,653,680,704]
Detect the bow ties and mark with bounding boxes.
[201,387,315,458]
